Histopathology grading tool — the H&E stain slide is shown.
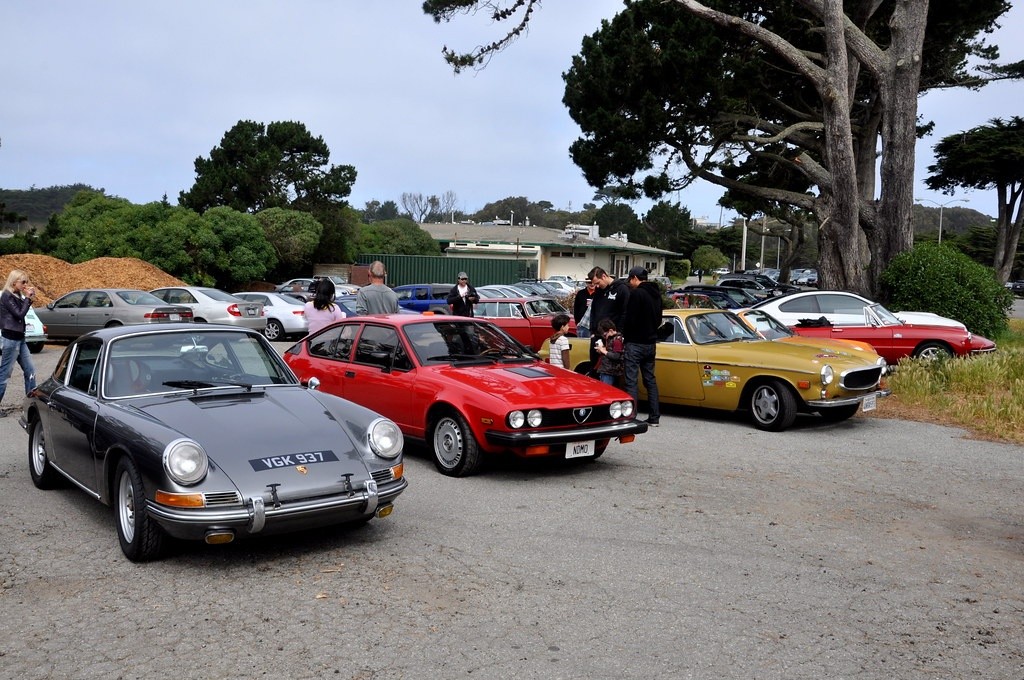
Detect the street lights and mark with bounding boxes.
[915,198,971,244]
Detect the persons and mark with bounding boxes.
[574,277,596,338]
[588,266,630,382]
[698,268,703,282]
[594,319,626,385]
[549,314,571,369]
[447,271,479,318]
[621,266,664,426]
[356,261,400,339]
[0,269,36,404]
[712,270,716,280]
[304,280,345,347]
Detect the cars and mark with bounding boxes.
[33,288,194,349]
[0,291,50,356]
[133,286,268,332]
[225,291,308,342]
[646,266,819,317]
[271,274,591,353]
[1003,279,1024,298]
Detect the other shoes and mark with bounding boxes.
[644,418,659,427]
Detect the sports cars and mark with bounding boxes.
[728,290,967,333]
[536,308,893,432]
[280,312,649,478]
[17,320,410,564]
[727,302,999,375]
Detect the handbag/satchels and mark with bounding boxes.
[579,300,592,330]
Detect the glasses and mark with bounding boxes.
[16,280,28,284]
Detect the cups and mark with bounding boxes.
[586,299,591,306]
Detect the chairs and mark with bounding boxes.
[93,358,149,397]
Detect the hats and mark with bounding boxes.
[623,266,647,282]
[458,272,468,279]
[583,276,592,282]
